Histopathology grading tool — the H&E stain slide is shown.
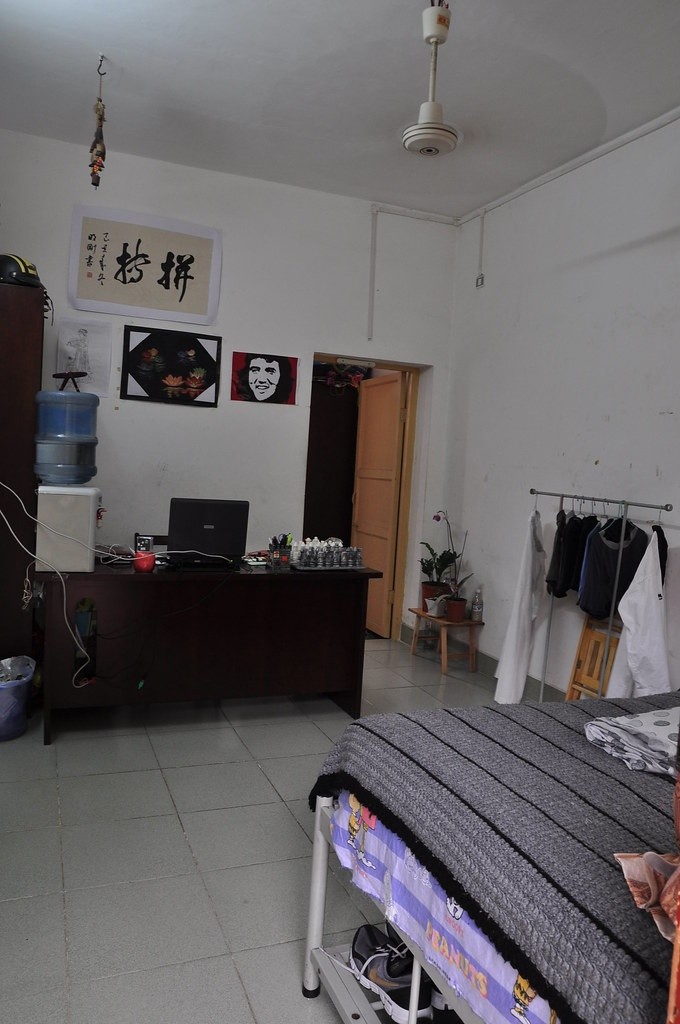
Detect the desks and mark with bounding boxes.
[36,548,383,745]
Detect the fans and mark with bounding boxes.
[403,0,456,161]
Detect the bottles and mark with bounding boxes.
[33,389,99,483]
[472,588,484,623]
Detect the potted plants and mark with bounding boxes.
[445,591,466,624]
[418,541,460,612]
[424,594,448,617]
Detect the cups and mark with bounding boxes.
[134,552,155,572]
[267,548,290,571]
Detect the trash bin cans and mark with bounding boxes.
[1,659,35,742]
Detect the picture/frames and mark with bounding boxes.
[65,201,225,325]
[116,322,225,410]
[53,316,120,399]
[228,349,300,408]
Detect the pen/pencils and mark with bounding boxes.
[268,533,292,546]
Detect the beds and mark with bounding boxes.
[302,686,678,1024]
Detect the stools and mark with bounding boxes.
[407,606,483,678]
